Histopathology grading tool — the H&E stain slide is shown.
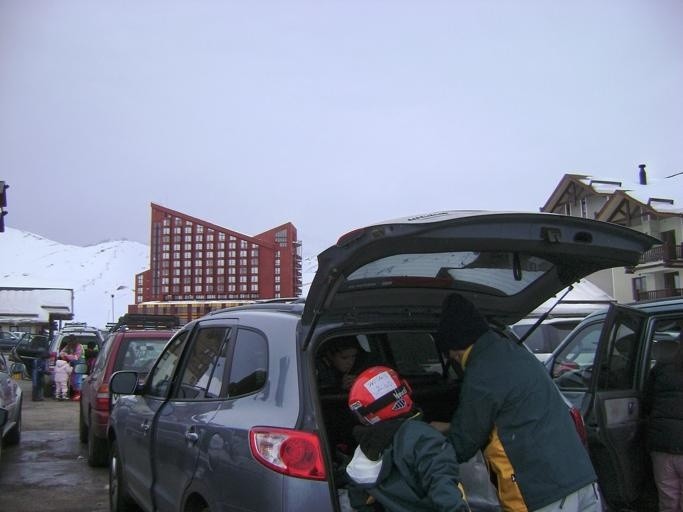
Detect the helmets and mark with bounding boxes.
[347,364,413,425]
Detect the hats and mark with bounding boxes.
[437,294,490,351]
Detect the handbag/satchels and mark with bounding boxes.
[74,363,88,374]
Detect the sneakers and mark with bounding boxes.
[56,394,69,400]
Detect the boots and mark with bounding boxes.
[32,385,45,401]
[70,390,80,400]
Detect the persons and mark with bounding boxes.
[29,351,51,402]
[346,366,469,512]
[85,341,96,358]
[429,289,603,512]
[53,353,73,399]
[642,331,682,511]
[311,333,369,396]
[58,335,88,401]
